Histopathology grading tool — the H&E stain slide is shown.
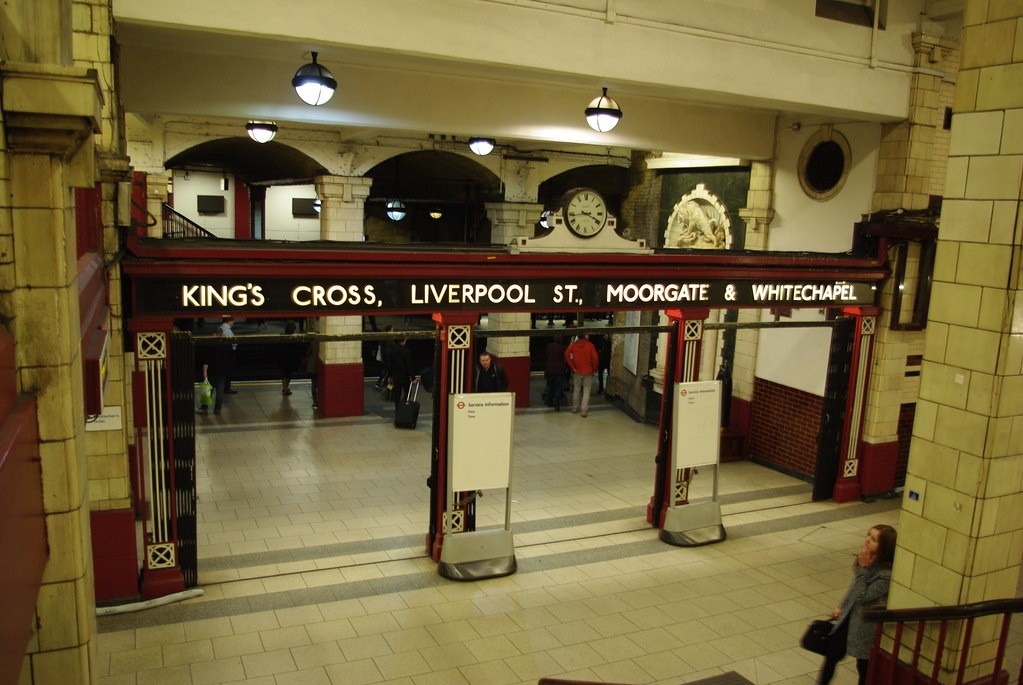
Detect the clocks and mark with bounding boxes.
[560,188,608,239]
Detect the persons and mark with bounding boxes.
[816,524,897,685]
[362,316,380,331]
[257,318,269,330]
[220,318,238,394]
[388,339,418,407]
[195,328,232,413]
[471,352,507,392]
[565,335,598,417]
[306,341,319,409]
[374,326,393,389]
[543,354,568,410]
[282,326,297,395]
[597,341,611,392]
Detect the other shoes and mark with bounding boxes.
[283,389,292,395]
[573,412,577,414]
[581,413,588,417]
[214,410,220,415]
[196,410,208,415]
[225,390,238,394]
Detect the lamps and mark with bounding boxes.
[540,179,562,228]
[313,200,321,212]
[385,156,406,221]
[583,87,623,134]
[292,51,337,107]
[468,137,496,156]
[429,205,442,219]
[245,120,278,143]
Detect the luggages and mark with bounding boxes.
[394,381,420,428]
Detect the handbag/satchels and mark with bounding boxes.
[801,618,839,657]
